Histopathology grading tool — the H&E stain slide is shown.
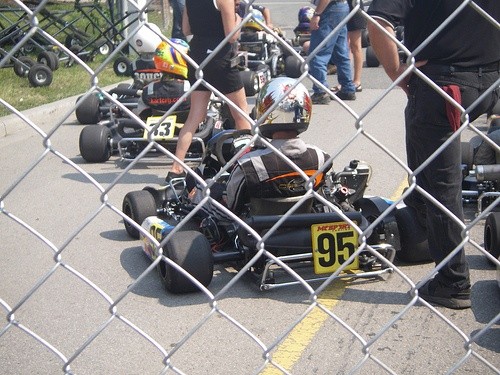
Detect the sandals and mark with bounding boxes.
[356,84,362,92]
[330,84,339,91]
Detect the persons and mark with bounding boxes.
[242,9,278,44]
[366,0,500,309]
[133,23,163,80]
[294,6,315,34]
[309,0,355,104]
[290,98,304,121]
[330,0,366,92]
[168,0,189,43]
[186,77,333,212]
[166,0,252,182]
[235,0,275,31]
[137,38,218,115]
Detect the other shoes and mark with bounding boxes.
[418,278,472,309]
[166,169,188,184]
[403,188,428,227]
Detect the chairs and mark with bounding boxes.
[118,31,500,247]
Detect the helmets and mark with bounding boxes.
[298,6,315,22]
[135,23,163,52]
[258,78,312,139]
[244,10,267,31]
[155,38,190,78]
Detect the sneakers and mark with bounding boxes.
[333,91,356,100]
[311,93,330,104]
